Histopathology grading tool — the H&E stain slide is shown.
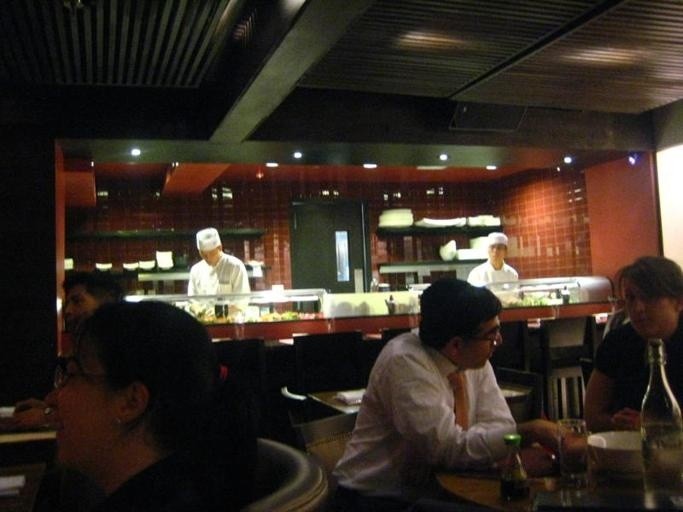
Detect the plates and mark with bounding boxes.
[377,208,502,263]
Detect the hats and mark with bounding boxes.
[195,227,221,252]
[486,232,509,248]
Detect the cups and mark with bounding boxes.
[556,418,590,490]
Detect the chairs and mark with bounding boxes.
[541,315,599,417]
[288,411,358,471]
[209,307,539,415]
[241,438,335,510]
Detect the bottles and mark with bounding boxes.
[500,434,528,502]
[640,338,683,512]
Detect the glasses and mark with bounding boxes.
[53,361,128,391]
[459,325,501,348]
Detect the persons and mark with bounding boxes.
[43,298,258,511]
[0,270,127,468]
[326,277,593,511]
[186,225,251,324]
[581,257,683,435]
[466,231,519,307]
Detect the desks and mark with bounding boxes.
[434,421,645,509]
[0,403,63,508]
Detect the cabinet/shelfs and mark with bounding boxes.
[379,215,502,293]
[77,229,270,305]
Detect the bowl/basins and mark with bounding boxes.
[95,251,174,273]
[587,430,643,483]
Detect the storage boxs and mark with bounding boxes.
[325,288,422,318]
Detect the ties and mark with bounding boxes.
[448,370,471,431]
